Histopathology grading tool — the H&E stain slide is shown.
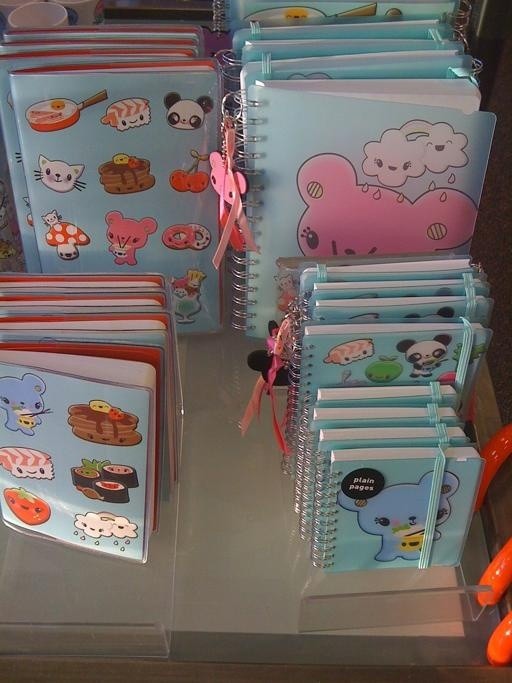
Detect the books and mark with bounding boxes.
[0,0,497,340]
[1,270,183,564]
[287,256,494,571]
